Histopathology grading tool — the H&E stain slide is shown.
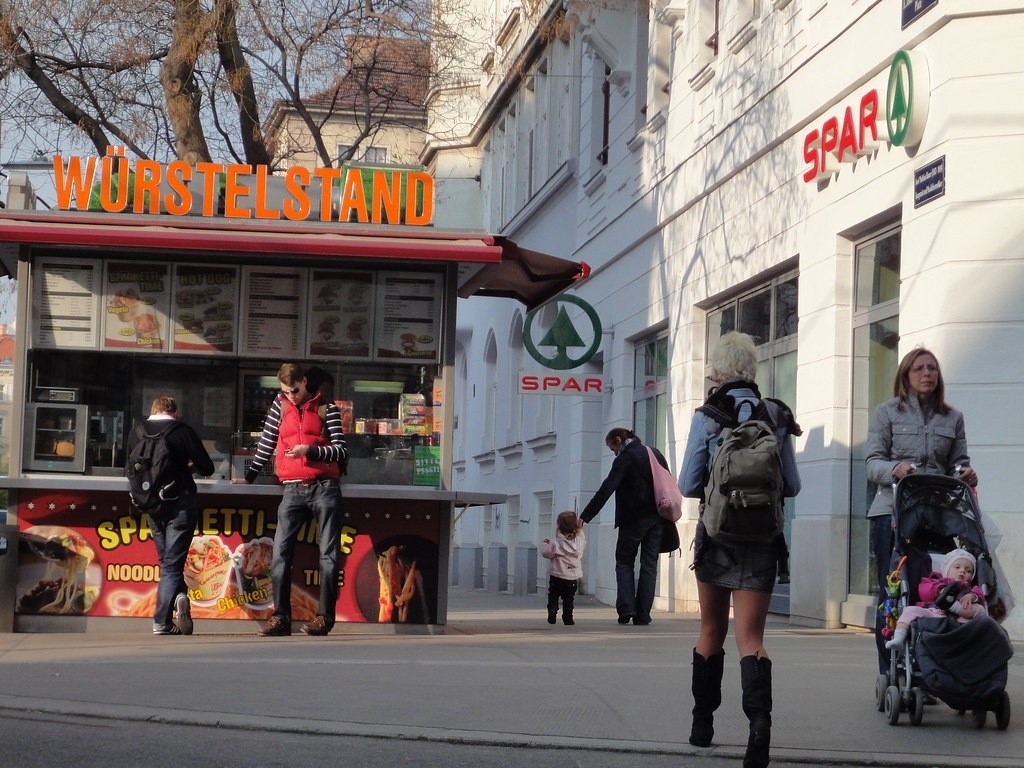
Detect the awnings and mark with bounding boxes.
[455,230,586,320]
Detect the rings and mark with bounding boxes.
[969,478,972,483]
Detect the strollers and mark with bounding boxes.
[872,466,1015,733]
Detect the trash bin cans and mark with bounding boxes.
[0,523,20,634]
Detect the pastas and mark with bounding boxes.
[377,545,417,623]
[17,524,274,616]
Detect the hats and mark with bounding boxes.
[941,549,976,583]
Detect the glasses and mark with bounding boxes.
[281,384,302,394]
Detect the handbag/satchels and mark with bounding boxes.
[645,445,682,523]
[658,522,681,553]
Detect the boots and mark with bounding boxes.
[688,646,727,748]
[739,655,773,768]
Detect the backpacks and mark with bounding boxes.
[694,400,784,544]
[124,420,186,516]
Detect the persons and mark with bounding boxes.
[578,427,672,626]
[677,329,806,768]
[864,346,980,690]
[884,548,989,649]
[125,395,217,637]
[541,509,586,627]
[230,360,352,638]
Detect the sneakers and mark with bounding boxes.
[173,593,194,635]
[153,619,182,635]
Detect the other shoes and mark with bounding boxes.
[561,613,575,625]
[617,615,631,624]
[257,617,293,637]
[632,616,651,625]
[547,611,557,625]
[300,615,336,636]
